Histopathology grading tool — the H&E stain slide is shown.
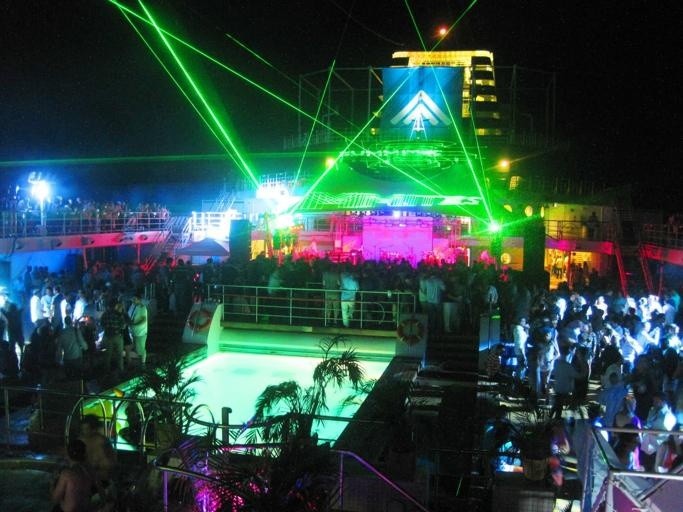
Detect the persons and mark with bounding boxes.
[585,210,600,242]
[0,253,153,403]
[2,193,169,236]
[152,250,463,334]
[47,440,99,512]
[78,412,121,512]
[464,258,683,512]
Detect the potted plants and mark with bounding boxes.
[240,333,361,509]
[489,374,591,480]
